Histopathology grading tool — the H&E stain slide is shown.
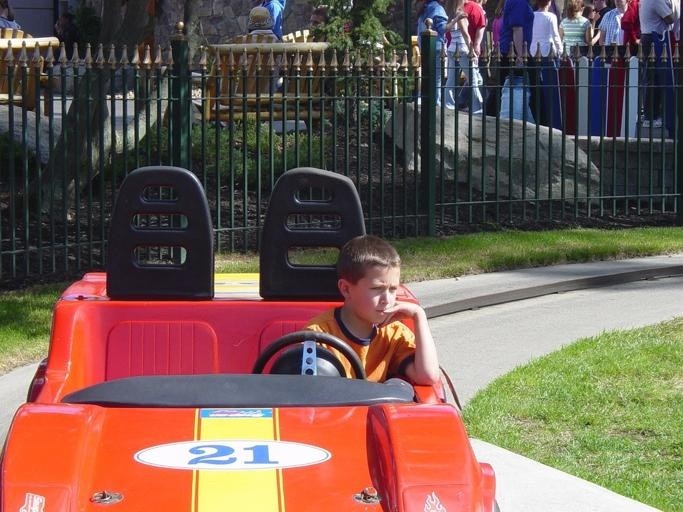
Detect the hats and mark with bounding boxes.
[247,6,272,30]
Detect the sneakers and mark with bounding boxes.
[643,120,662,128]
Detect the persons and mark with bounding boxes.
[309,4,332,27]
[417,0,683,139]
[247,0,286,92]
[301,235,440,386]
[0,0,21,30]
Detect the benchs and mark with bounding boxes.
[202,30,333,126]
[0,28,60,116]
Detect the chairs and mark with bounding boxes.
[104,318,220,381]
[259,319,313,379]
